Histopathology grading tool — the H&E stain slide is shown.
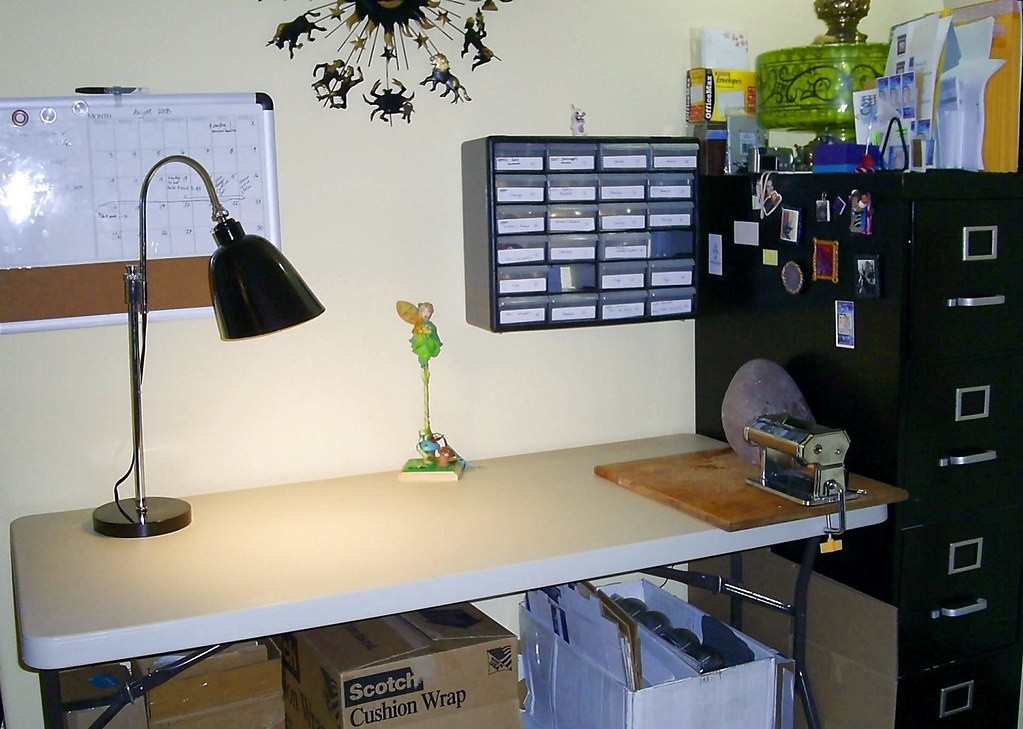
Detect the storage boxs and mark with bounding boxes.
[270,602,521,729]
[519,580,798,729]
[56,635,285,729]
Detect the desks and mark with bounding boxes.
[10,434,909,729]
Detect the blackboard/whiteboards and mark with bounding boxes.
[0,91,279,336]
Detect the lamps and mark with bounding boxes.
[94,155,323,540]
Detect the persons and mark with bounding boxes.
[838,313,850,333]
[763,175,774,199]
[850,192,872,235]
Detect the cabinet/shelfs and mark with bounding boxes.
[691,169,1023,729]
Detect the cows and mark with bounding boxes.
[266,12,327,59]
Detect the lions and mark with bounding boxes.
[419,52,472,105]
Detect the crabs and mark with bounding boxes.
[362,77,415,124]
